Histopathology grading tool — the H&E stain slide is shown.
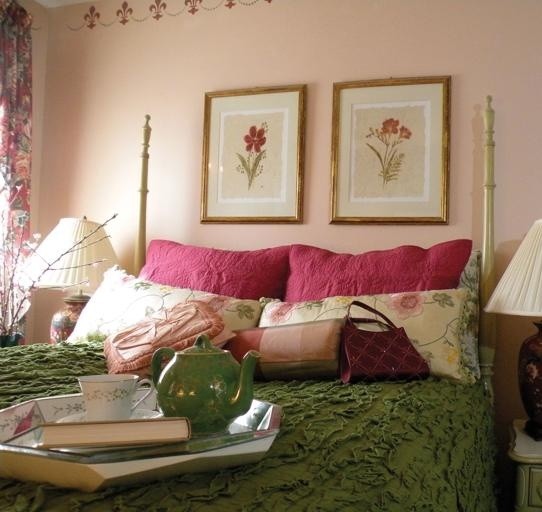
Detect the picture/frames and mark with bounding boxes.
[328,75,451,226]
[199,84,305,223]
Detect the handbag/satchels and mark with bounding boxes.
[340,301,430,384]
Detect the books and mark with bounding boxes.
[41,418,192,449]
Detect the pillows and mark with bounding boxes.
[461,251,483,388]
[286,238,473,303]
[139,240,291,304]
[105,299,235,376]
[223,318,343,378]
[259,289,478,386]
[66,265,280,355]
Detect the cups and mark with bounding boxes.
[76,370,152,420]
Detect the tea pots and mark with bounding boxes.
[150,333,261,434]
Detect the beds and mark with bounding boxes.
[0,94,498,512]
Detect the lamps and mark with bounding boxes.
[483,220,542,440]
[15,218,120,344]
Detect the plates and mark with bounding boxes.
[55,408,160,424]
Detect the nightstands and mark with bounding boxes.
[508,417,542,511]
